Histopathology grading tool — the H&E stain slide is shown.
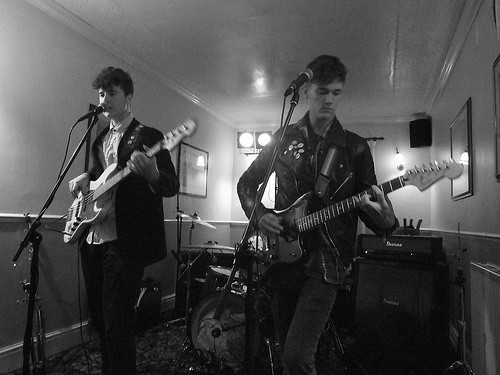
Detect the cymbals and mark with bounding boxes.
[181,244,239,250]
[170,211,217,232]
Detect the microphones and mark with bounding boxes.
[212,329,219,337]
[205,250,217,263]
[76,102,109,123]
[171,250,186,269]
[284,69,313,97]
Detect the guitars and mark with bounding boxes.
[238,159,463,283]
[63,121,198,242]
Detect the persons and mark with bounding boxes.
[68,67,179,375]
[236,55,399,375]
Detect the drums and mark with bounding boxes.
[204,266,239,289]
[189,293,246,372]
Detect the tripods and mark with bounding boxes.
[444,249,476,375]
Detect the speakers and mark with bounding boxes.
[349,258,452,370]
[410,119,432,148]
